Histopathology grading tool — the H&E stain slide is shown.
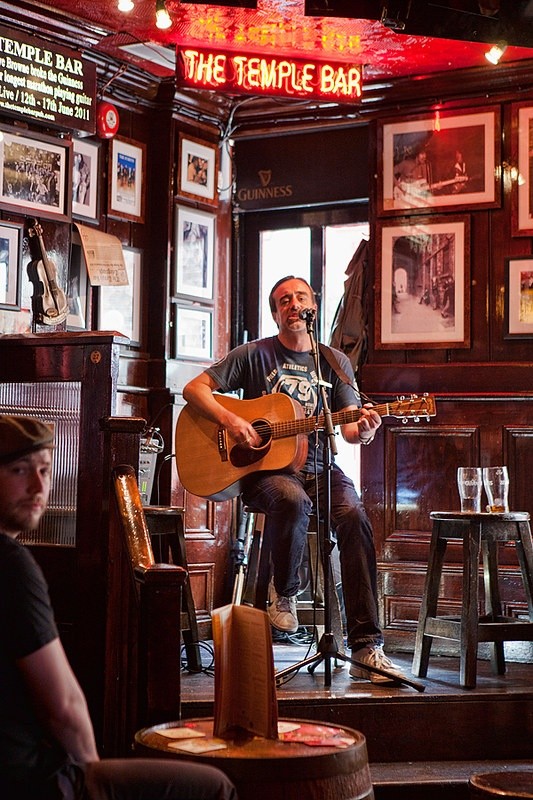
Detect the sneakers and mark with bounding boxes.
[349,647,404,683]
[267,576,298,633]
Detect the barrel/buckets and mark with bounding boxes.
[130,717,374,800]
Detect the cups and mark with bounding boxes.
[482,466,509,513]
[457,467,482,513]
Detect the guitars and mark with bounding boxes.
[394,176,469,200]
[175,393,437,502]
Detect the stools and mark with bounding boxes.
[412,511,533,691]
[241,507,345,668]
[142,505,202,675]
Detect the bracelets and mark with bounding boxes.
[358,436,374,446]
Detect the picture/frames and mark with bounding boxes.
[374,101,533,349]
[0,121,219,362]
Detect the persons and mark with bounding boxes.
[72,153,90,203]
[0,414,101,762]
[188,157,208,185]
[182,276,406,682]
[442,146,469,194]
[393,144,442,194]
[117,163,132,187]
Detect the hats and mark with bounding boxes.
[0,414,56,467]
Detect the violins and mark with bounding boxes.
[28,218,67,318]
